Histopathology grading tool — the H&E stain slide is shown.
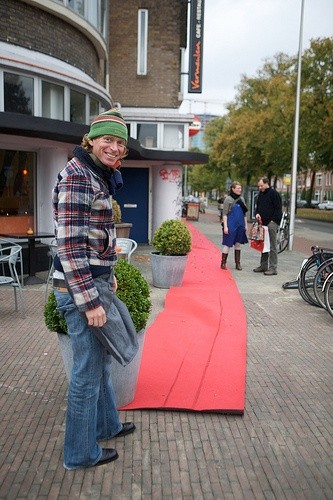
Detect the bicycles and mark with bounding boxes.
[275,197,290,254]
[282,244,333,318]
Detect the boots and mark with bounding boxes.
[220,253,227,270]
[234,249,242,270]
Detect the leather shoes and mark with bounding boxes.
[115,422,134,437]
[93,449,120,465]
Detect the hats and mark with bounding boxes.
[87,107,127,143]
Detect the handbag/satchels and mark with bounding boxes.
[83,272,140,366]
[247,218,263,242]
[250,217,271,253]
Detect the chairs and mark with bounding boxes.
[0,237,25,289]
[115,236,138,267]
[0,245,27,321]
[45,240,61,308]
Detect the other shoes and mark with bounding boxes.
[253,265,266,271]
[264,269,277,275]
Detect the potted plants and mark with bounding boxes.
[43,259,153,409]
[112,199,134,242]
[150,218,192,288]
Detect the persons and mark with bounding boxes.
[221,181,248,270]
[253,177,283,276]
[54,106,140,471]
